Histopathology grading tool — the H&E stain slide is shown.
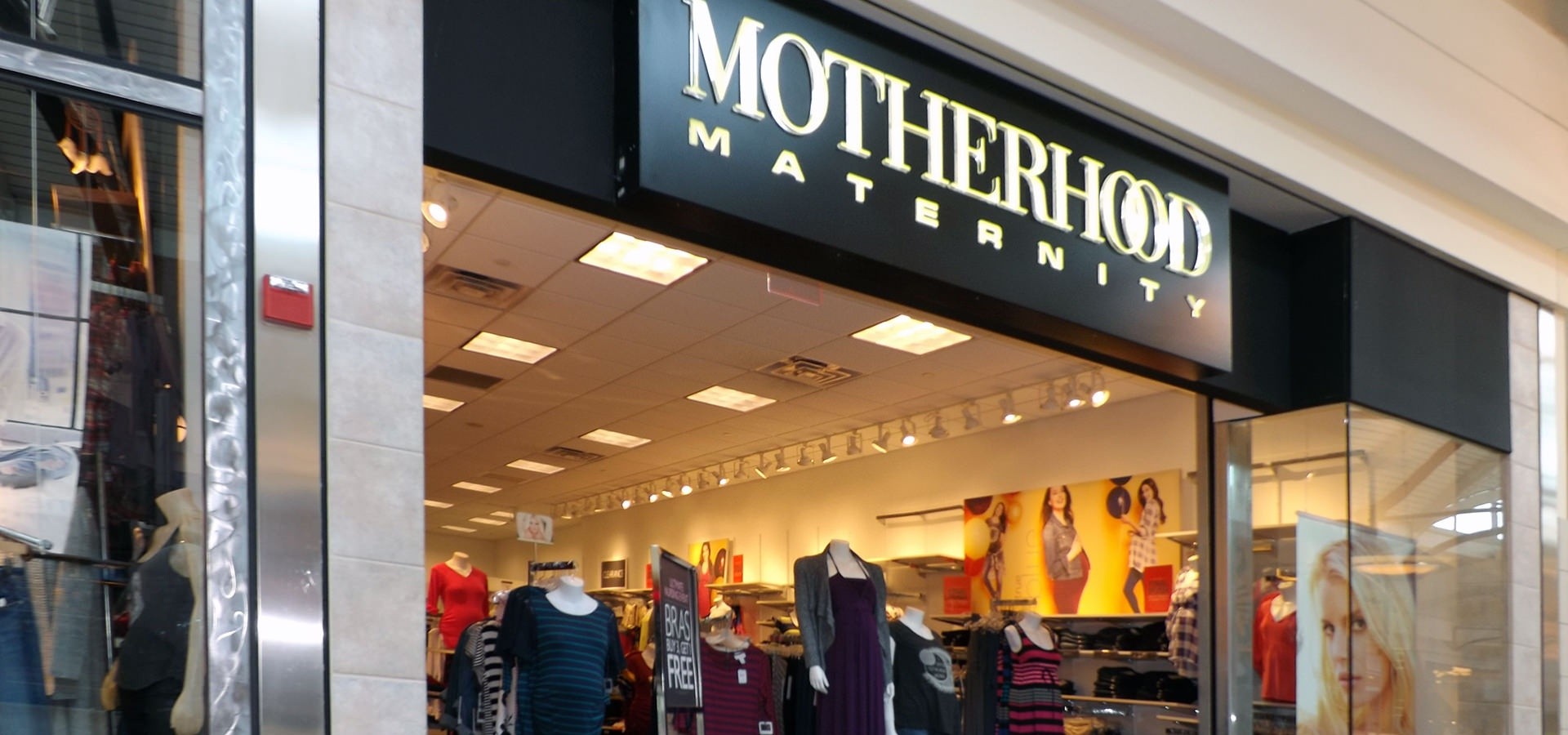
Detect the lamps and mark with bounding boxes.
[423,190,461,231]
[551,368,1110,519]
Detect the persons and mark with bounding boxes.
[511,573,626,735]
[521,516,549,543]
[1119,477,1165,612]
[1165,552,1199,676]
[977,502,1012,608]
[1037,484,1091,613]
[104,487,207,735]
[792,538,892,734]
[1250,580,1299,704]
[638,600,655,648]
[1307,537,1418,734]
[710,597,734,635]
[428,551,492,651]
[695,542,717,618]
[627,642,657,734]
[879,606,962,735]
[1004,612,1068,734]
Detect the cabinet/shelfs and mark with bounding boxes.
[584,519,1373,725]
